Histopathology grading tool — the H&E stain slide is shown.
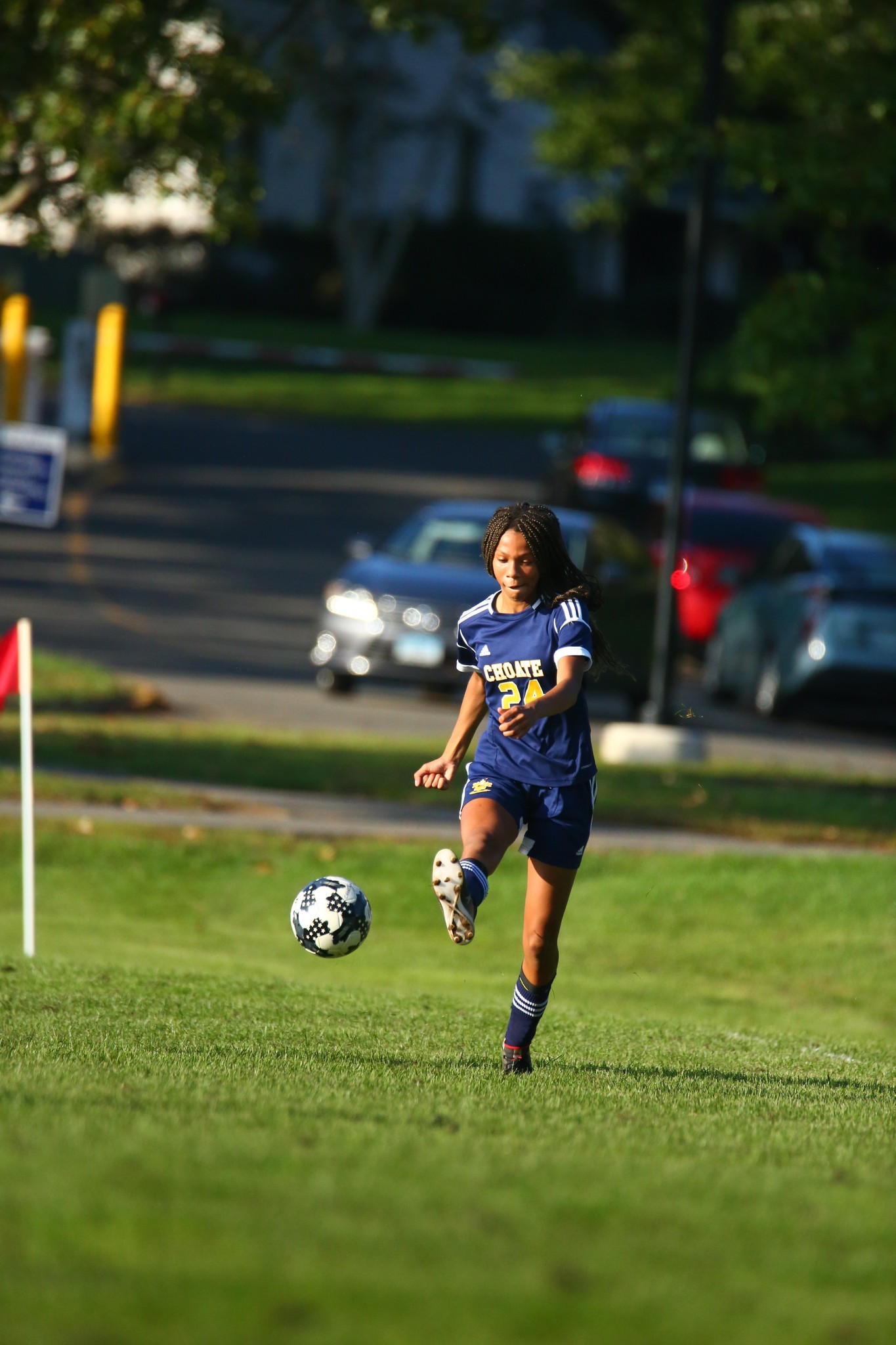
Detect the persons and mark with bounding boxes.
[411,502,599,1080]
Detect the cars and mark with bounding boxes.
[537,390,765,535]
[308,501,658,711]
[700,524,896,727]
[578,485,825,649]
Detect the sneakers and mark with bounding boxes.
[430,845,477,946]
[502,1040,533,1075]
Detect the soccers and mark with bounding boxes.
[290,875,372,959]
